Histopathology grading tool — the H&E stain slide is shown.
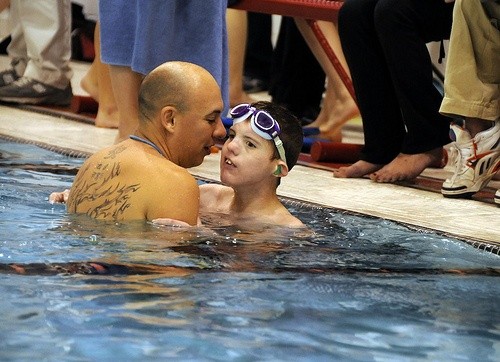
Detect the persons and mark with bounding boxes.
[48,101,308,242]
[0,0,500,204]
[66,60,227,225]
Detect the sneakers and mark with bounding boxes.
[441,120,500,197]
[494,189,500,204]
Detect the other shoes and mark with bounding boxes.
[303,137,342,151]
[0,77,73,107]
[302,125,322,134]
[0,67,22,87]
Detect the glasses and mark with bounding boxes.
[230,103,287,162]
